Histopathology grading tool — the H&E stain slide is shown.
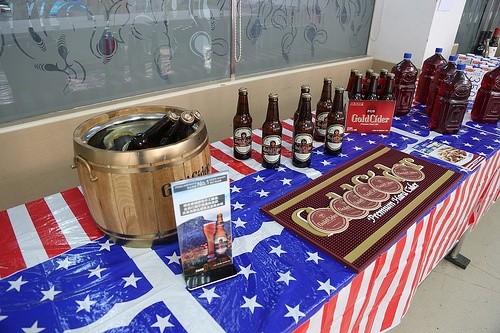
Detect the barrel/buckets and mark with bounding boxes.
[70,105,212,248]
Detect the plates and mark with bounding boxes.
[430,146,473,165]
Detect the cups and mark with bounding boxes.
[203,222,217,258]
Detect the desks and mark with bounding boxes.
[0,80,500,333]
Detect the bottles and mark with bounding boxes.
[293,84,311,124]
[292,94,314,168]
[213,214,228,263]
[456,27,500,122]
[428,62,472,134]
[233,86,253,159]
[391,52,418,117]
[344,68,396,112]
[415,47,447,105]
[261,93,283,169]
[313,77,333,143]
[425,55,458,116]
[121,111,202,151]
[323,86,346,156]
